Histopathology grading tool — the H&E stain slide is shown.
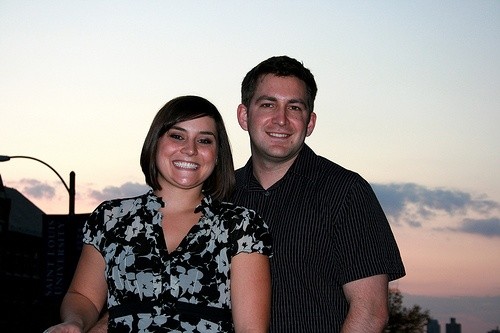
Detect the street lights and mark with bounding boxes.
[0,154,76,216]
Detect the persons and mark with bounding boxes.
[86,55,408,332]
[42,95,278,332]
